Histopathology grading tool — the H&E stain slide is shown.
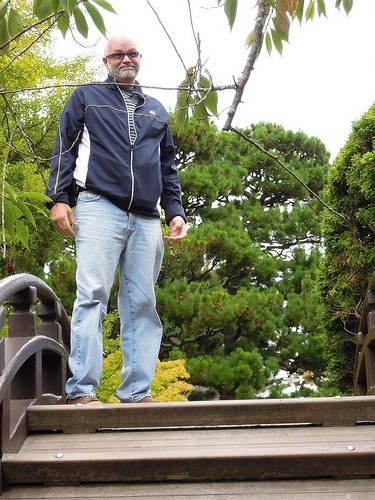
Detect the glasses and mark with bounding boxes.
[106,51,139,60]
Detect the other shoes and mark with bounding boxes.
[138,397,158,404]
[67,395,101,405]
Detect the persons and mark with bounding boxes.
[42,34,189,404]
